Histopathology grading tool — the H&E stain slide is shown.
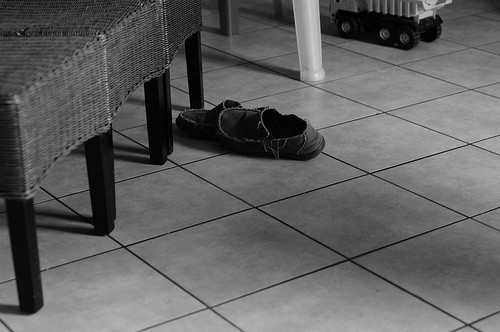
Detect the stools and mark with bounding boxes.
[0,35,115,315]
[0,0,167,221]
[160,1,206,155]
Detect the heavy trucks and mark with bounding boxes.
[329,0,454,51]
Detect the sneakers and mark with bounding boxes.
[219,106,325,160]
[175,99,245,141]
